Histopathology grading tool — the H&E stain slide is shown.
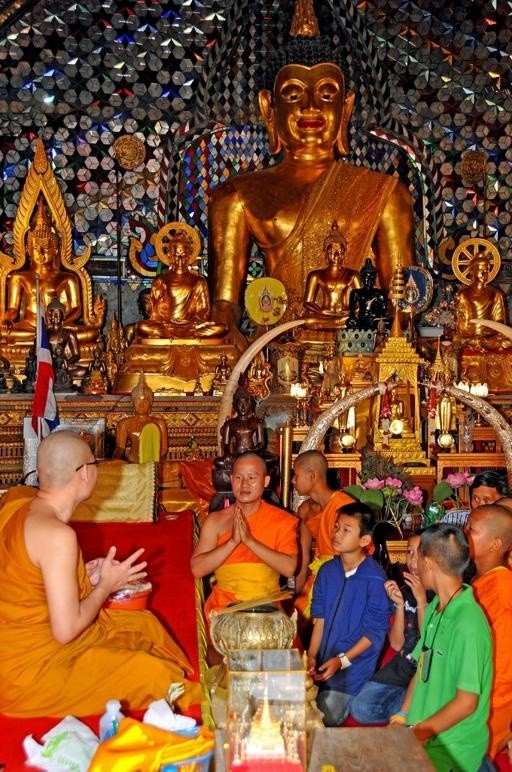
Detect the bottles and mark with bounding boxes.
[100,700,125,743]
[428,499,440,522]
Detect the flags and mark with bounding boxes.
[26,275,61,442]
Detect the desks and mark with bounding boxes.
[386,540,410,564]
[291,453,362,485]
[395,468,437,509]
[214,727,436,772]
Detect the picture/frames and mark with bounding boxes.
[25,418,104,459]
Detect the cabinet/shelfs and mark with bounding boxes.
[278,424,321,477]
[0,392,222,486]
[437,427,511,509]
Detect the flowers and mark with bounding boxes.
[343,477,424,527]
[432,471,476,504]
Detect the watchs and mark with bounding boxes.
[335,651,353,671]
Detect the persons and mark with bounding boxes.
[134,234,229,338]
[323,338,342,393]
[214,372,277,468]
[385,523,492,771]
[0,223,99,345]
[452,251,512,350]
[1,430,206,716]
[290,502,391,725]
[347,259,394,331]
[380,388,410,423]
[106,351,118,382]
[24,296,89,378]
[124,289,152,344]
[211,348,232,384]
[350,532,434,724]
[301,219,363,330]
[458,504,511,765]
[112,368,169,463]
[286,450,375,638]
[436,472,511,524]
[210,0,419,355]
[83,344,108,379]
[190,454,301,666]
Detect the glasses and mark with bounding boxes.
[75,459,100,471]
